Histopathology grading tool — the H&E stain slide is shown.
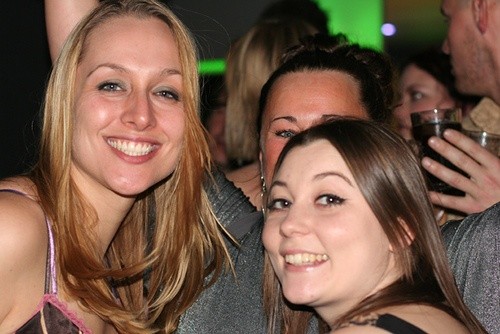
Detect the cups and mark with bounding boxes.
[450,133,500,216]
[411,107,463,195]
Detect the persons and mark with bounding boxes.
[43,1,500,226]
[169,41,498,334]
[1,1,242,333]
[262,113,488,333]
